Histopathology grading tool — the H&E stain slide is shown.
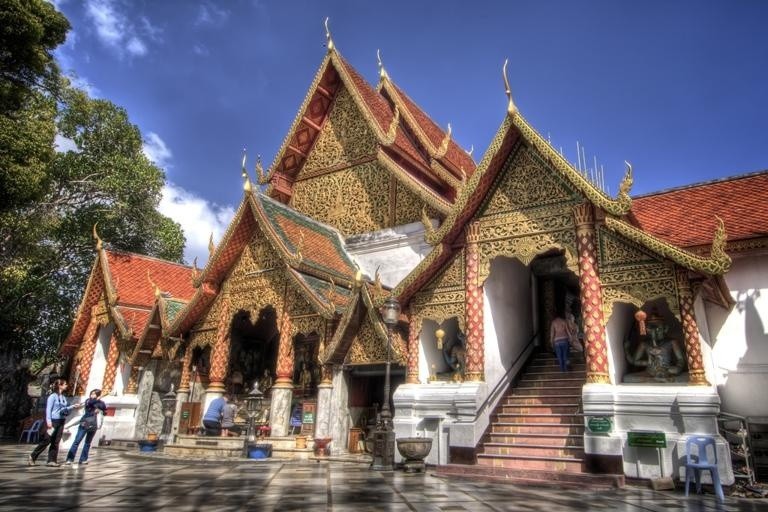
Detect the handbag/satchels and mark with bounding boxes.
[96,410,104,429]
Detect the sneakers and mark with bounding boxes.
[47,462,59,467]
[28,456,35,466]
[197,429,207,436]
[65,460,72,465]
[81,460,89,464]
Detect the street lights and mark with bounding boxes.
[368,293,401,431]
[247,381,264,442]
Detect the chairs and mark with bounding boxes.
[680,433,728,502]
[18,417,45,445]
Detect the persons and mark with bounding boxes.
[629,304,684,380]
[444,324,469,382]
[299,363,312,384]
[260,367,272,393]
[28,377,81,468]
[196,393,231,434]
[222,397,242,437]
[550,312,573,371]
[66,388,107,464]
